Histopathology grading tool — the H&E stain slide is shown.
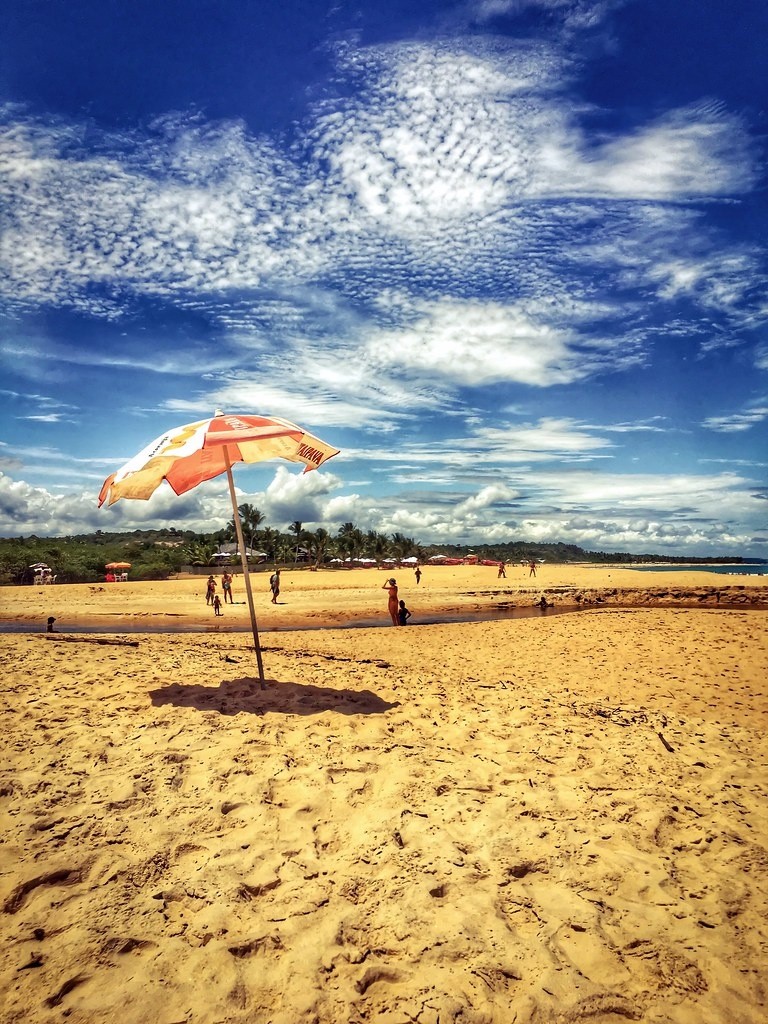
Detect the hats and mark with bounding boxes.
[48,617,56,620]
[208,576,214,578]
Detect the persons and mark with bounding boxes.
[214,596,222,615]
[205,575,217,605]
[529,562,537,577]
[42,573,52,585]
[231,569,238,577]
[497,562,507,578]
[269,570,282,604]
[221,571,234,603]
[398,600,411,626]
[381,578,399,626]
[414,568,422,584]
[108,570,123,576]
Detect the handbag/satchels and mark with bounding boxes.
[270,575,274,584]
[225,583,229,589]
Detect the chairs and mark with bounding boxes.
[121,573,128,581]
[35,575,57,585]
[114,574,122,582]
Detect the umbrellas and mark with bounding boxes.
[105,561,132,572]
[330,555,502,567]
[98,408,341,690]
[29,562,48,576]
